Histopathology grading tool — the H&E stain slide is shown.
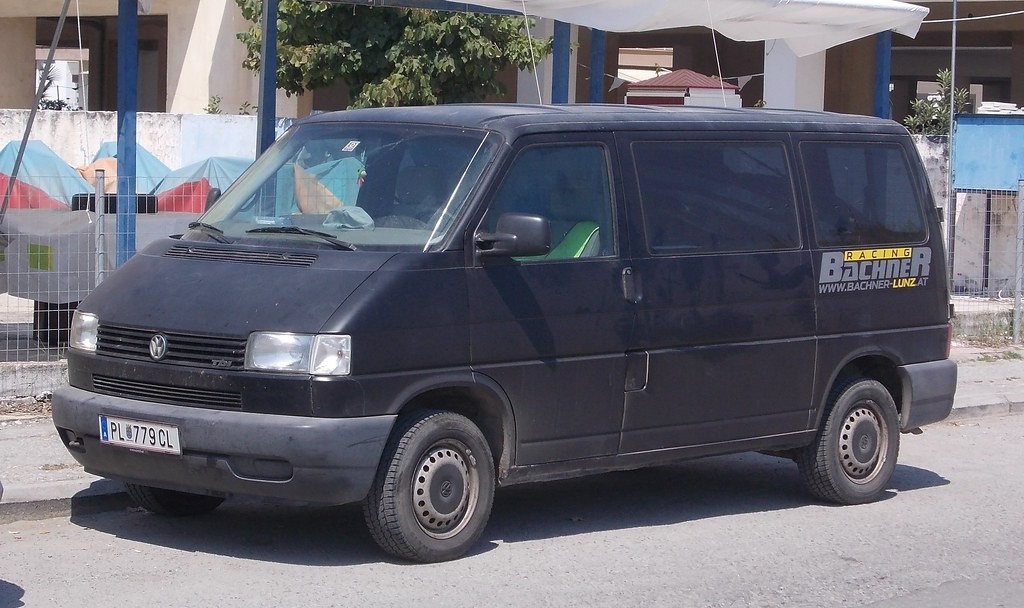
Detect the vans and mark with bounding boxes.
[50,100,957,561]
